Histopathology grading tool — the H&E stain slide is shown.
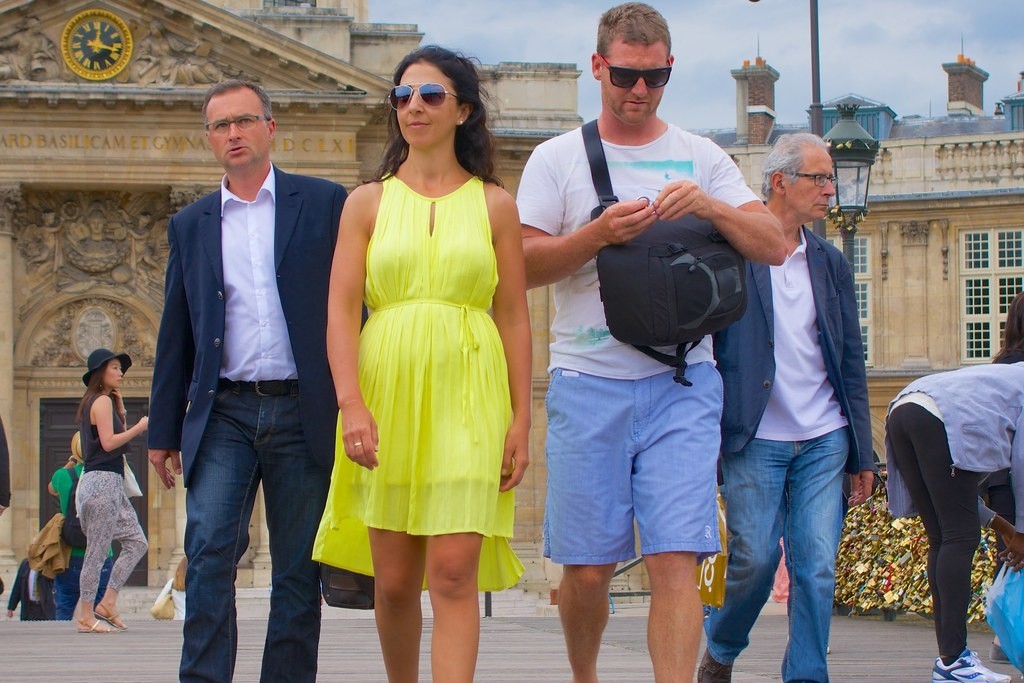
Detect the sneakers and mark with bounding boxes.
[932,649,1014,683]
[698,649,731,683]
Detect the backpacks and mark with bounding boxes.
[63,465,88,547]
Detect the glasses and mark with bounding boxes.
[206,116,268,135]
[796,174,837,188]
[389,82,457,111]
[600,54,672,88]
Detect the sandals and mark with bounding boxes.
[94,604,128,631]
[77,619,118,633]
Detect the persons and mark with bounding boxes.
[697,131,873,683]
[311,45,533,683]
[74,348,149,633]
[48,428,116,621]
[884,290,1024,683]
[516,2,787,683]
[6,544,56,621]
[152,80,368,683]
[0,415,11,594]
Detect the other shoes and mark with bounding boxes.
[990,642,1013,665]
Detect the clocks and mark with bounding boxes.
[60,9,131,78]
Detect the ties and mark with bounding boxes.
[32,572,39,600]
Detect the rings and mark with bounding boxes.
[354,442,362,446]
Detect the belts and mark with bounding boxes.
[219,378,300,397]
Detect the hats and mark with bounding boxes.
[83,349,132,387]
[71,431,83,463]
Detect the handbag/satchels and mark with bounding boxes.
[987,562,1024,674]
[698,486,728,609]
[592,206,748,387]
[151,581,175,619]
[320,561,375,610]
[122,454,142,498]
